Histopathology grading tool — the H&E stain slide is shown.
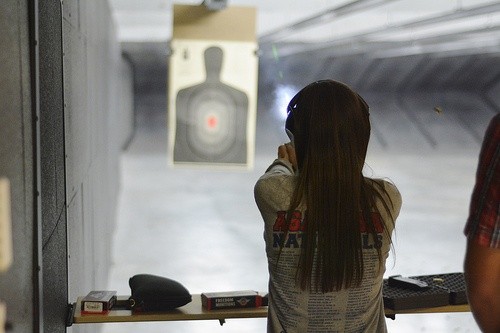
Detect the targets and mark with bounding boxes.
[165,39,260,166]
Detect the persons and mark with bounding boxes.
[254,79,402,333]
[463,110,500,333]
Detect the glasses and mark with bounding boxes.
[286,79,318,126]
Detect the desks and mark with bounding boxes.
[71,287,472,323]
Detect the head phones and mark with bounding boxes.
[284,91,301,144]
